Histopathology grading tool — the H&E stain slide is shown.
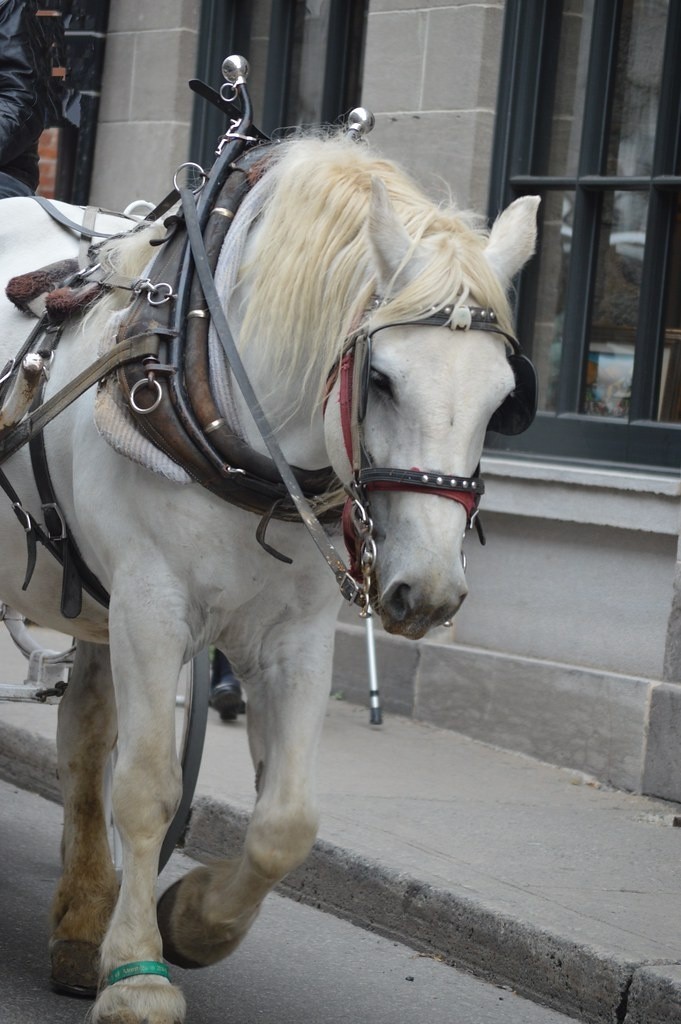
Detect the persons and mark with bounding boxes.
[209,647,246,719]
[0,0,49,199]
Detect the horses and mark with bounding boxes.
[0,131,542,1024]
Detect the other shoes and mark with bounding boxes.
[209,674,246,720]
